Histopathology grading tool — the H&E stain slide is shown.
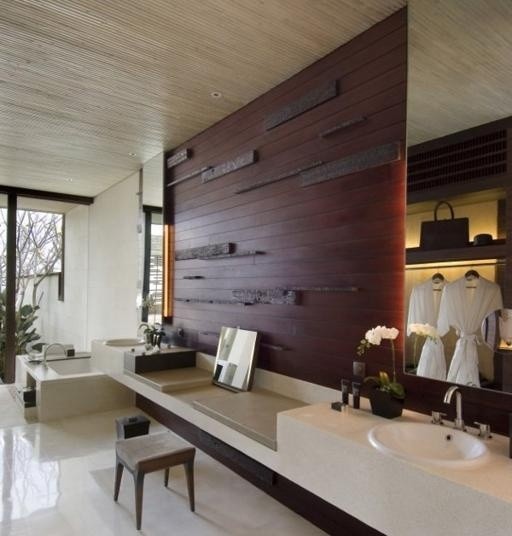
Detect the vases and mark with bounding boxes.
[152,333,162,346]
[367,387,403,419]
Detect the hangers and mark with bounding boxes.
[447,264,480,288]
[432,266,450,291]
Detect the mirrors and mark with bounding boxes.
[480,308,512,355]
[211,326,262,393]
[139,150,165,325]
[404,0,512,394]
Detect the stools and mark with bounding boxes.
[113,431,196,530]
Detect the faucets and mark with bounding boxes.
[443,385,465,433]
[135,323,151,345]
[43,341,68,362]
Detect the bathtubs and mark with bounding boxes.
[43,354,102,376]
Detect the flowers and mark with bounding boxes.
[357,325,407,400]
[139,292,165,335]
[406,322,440,374]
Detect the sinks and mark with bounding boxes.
[104,337,145,349]
[368,420,491,467]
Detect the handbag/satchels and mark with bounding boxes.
[419,218,469,250]
